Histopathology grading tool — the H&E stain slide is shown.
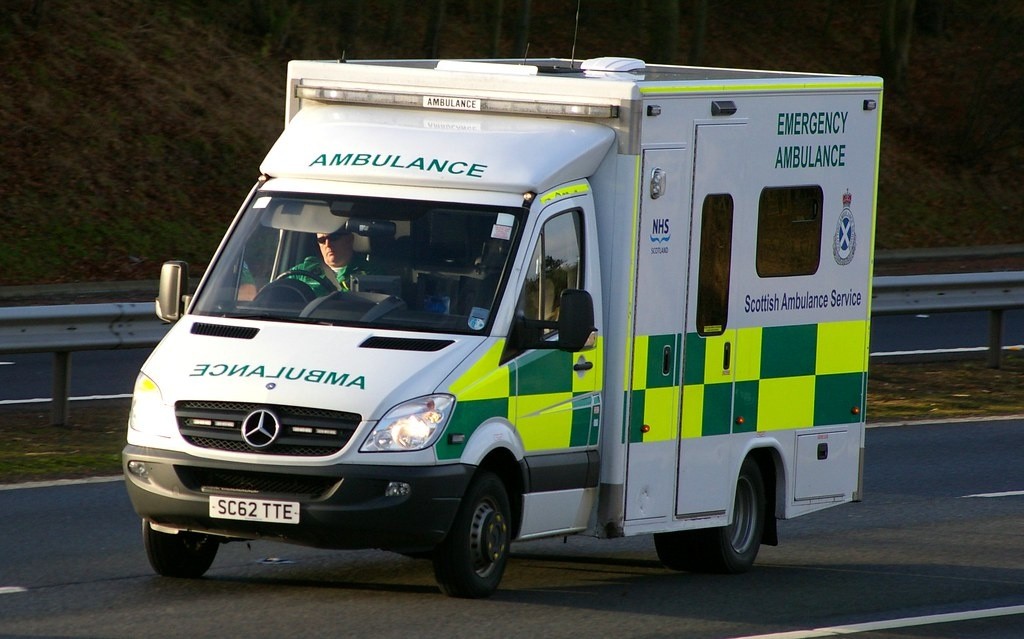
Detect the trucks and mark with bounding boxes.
[122,54,884,598]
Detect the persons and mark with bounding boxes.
[222,243,256,303]
[280,213,377,301]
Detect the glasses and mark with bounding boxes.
[317,233,337,243]
